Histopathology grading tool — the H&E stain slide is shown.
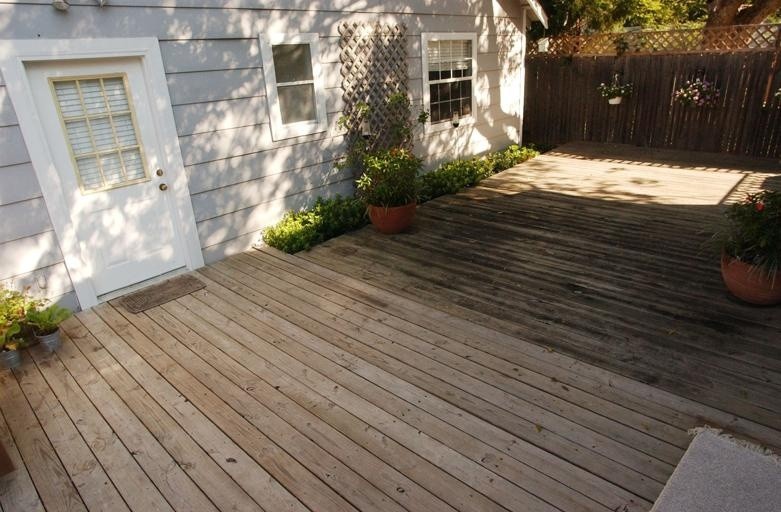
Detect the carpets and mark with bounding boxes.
[646,425,781,511]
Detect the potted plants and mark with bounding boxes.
[0,281,73,369]
[354,147,426,233]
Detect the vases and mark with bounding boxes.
[608,97,623,105]
[689,102,698,109]
[721,246,780,306]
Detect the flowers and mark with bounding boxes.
[670,75,721,110]
[695,189,781,291]
[597,72,634,98]
